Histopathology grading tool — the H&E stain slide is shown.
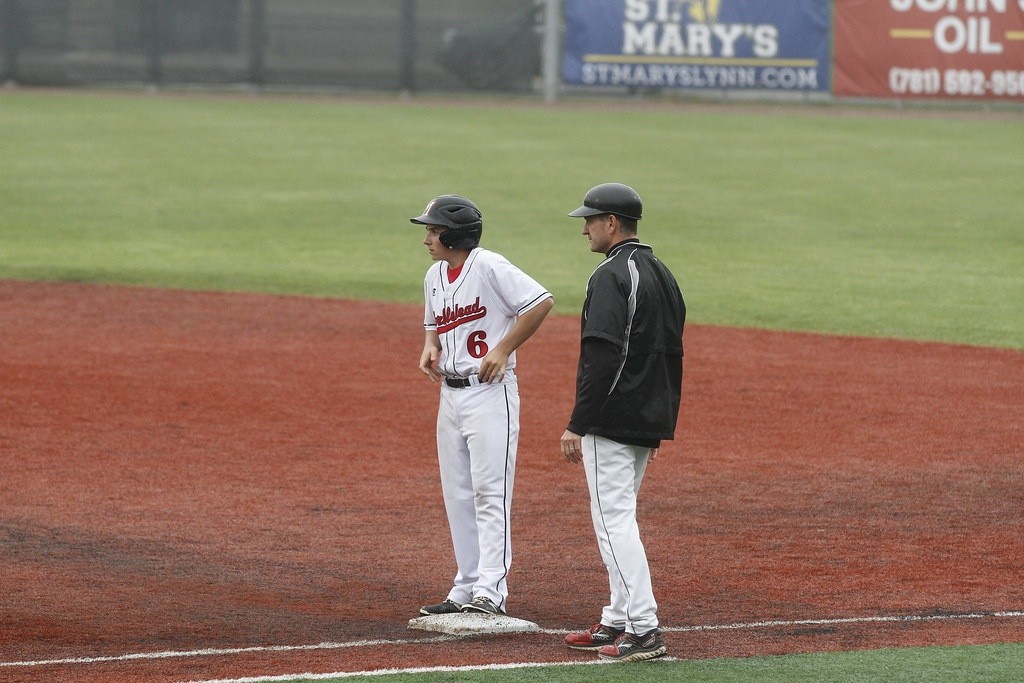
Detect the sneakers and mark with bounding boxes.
[565,624,625,650]
[460,596,504,615]
[420,599,463,616]
[598,629,667,662]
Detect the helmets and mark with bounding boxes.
[567,183,643,220]
[409,194,482,250]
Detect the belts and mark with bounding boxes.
[445,375,489,389]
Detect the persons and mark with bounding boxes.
[410,195,555,617]
[559,182,687,662]
